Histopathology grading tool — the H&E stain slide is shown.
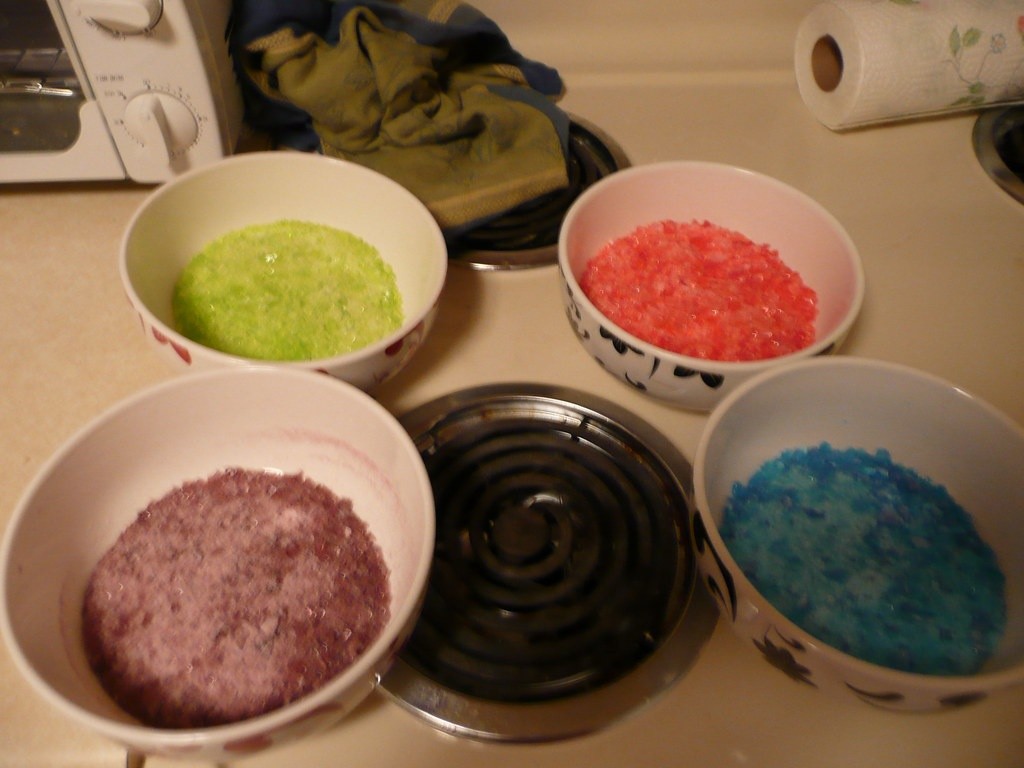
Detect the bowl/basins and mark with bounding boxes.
[557,159,866,415]
[689,354,1024,717]
[117,147,448,396]
[0,365,436,755]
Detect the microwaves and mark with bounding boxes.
[0,0,246,184]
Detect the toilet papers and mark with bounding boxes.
[794,0,1024,132]
